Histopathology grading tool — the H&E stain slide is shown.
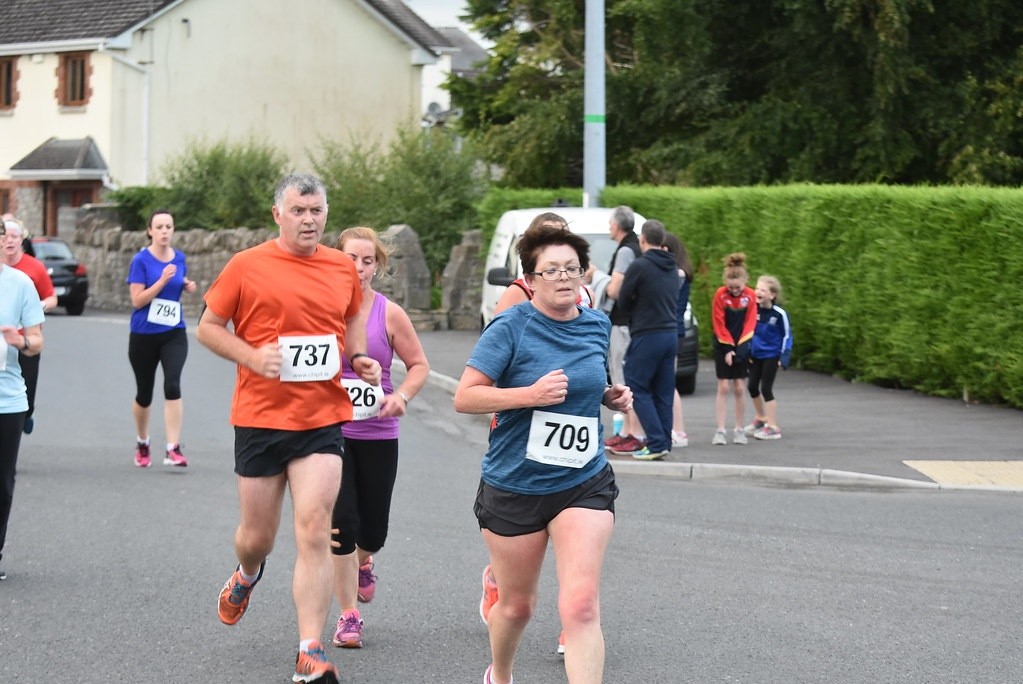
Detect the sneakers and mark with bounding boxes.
[671,431,688,447]
[712,431,726,445]
[483,663,513,684]
[733,430,748,445]
[334,610,364,648]
[744,419,767,434]
[610,435,648,454]
[480,565,501,624]
[292,640,338,684]
[754,424,781,439]
[164,444,187,466]
[633,446,668,459]
[604,435,626,449]
[135,439,152,468]
[558,631,567,654]
[357,555,378,601]
[218,557,266,625]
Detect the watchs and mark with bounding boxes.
[19,335,30,352]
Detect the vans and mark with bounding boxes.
[474,206,699,396]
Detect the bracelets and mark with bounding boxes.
[350,353,369,372]
[393,391,408,405]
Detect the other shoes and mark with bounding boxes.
[23,416,33,434]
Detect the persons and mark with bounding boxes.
[746,275,793,439]
[330,226,430,649]
[710,252,757,445]
[583,204,688,461]
[0,216,46,580]
[454,224,634,682]
[0,213,58,436]
[127,210,196,468]
[479,212,595,655]
[197,173,381,684]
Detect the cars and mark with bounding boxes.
[22,235,90,316]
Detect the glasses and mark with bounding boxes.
[529,266,584,281]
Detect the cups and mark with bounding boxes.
[613,412,623,436]
